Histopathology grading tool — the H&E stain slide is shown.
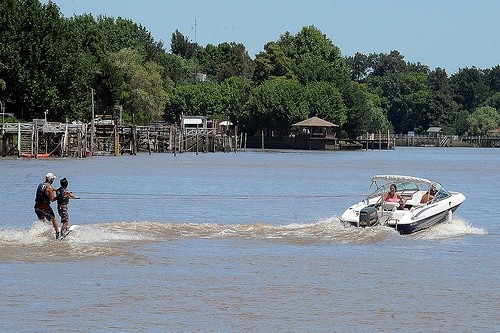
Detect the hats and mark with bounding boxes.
[45,172,57,181]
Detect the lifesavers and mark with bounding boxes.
[38,153,49,159]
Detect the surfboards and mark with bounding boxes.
[49,222,78,242]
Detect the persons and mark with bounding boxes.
[33,173,60,238]
[56,178,78,236]
[380,184,405,211]
[421,188,437,203]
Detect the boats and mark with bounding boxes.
[341,171,468,235]
